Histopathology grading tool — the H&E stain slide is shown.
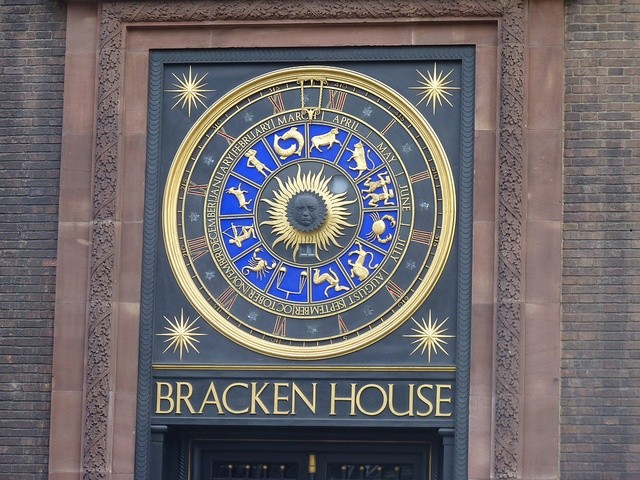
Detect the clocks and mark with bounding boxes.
[161,64,457,362]
